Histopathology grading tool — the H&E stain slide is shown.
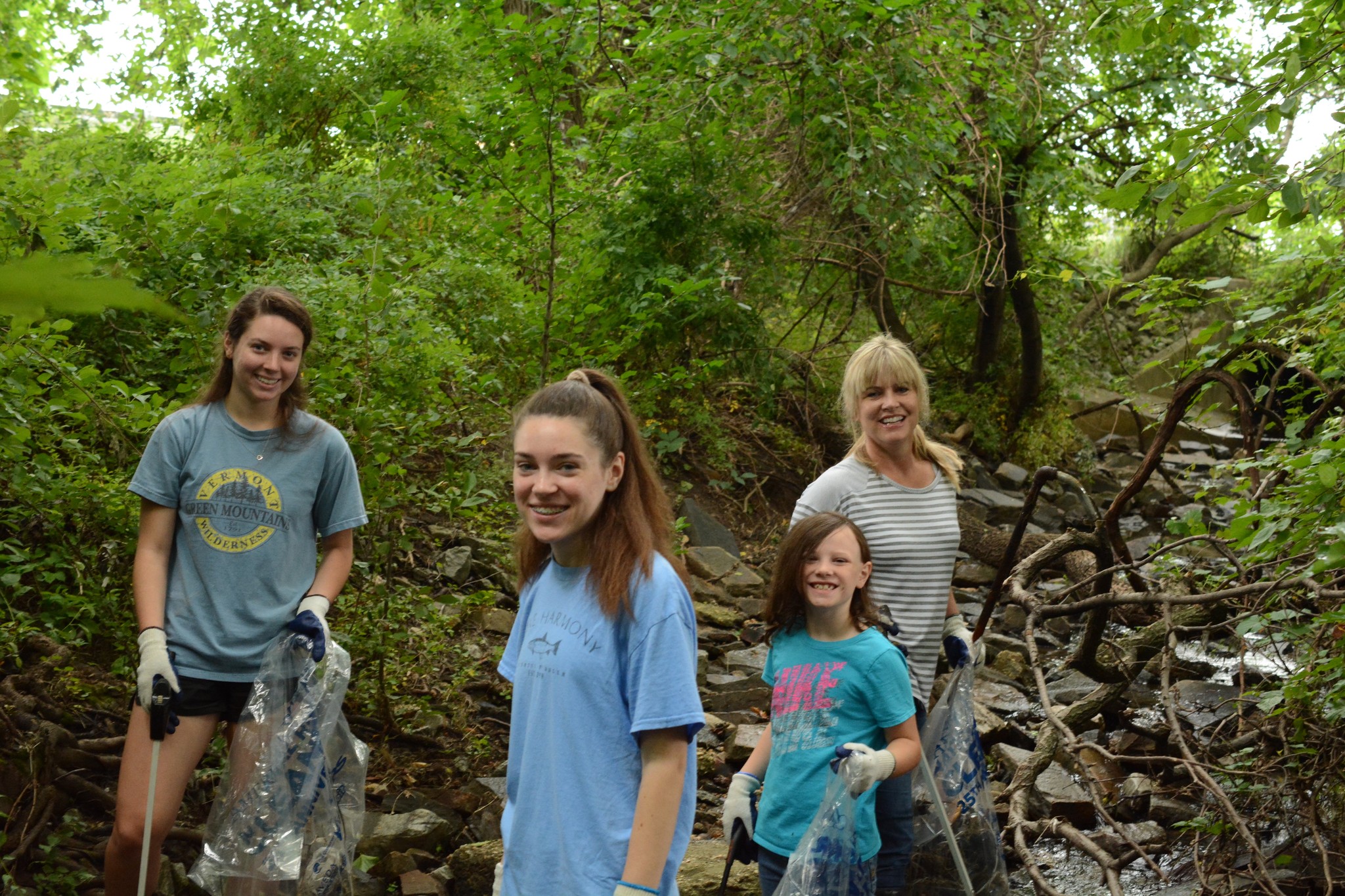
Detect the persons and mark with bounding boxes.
[496,367,708,896]
[102,286,370,896]
[788,335,988,896]
[725,513,923,896]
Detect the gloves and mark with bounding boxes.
[940,612,976,670]
[137,626,181,734]
[830,742,893,798]
[722,773,762,864]
[287,594,331,663]
